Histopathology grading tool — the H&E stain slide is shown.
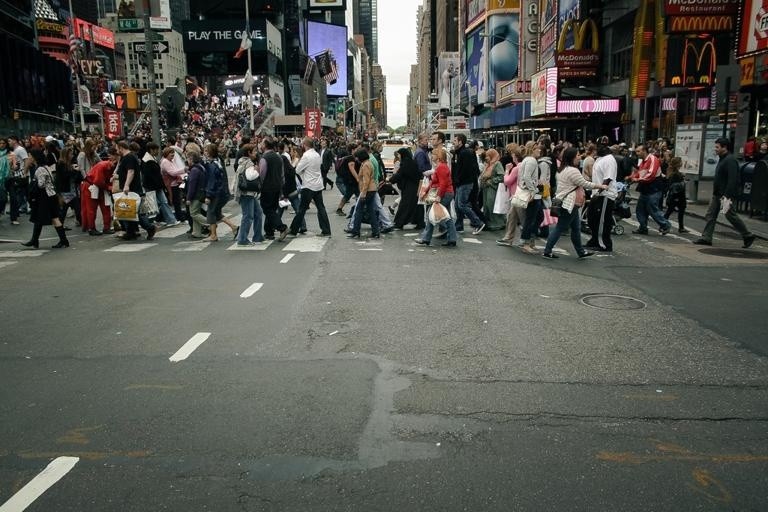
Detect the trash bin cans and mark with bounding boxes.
[736,161,768,221]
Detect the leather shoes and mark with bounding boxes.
[344,229,391,238]
[441,241,456,247]
[414,238,431,245]
[203,237,217,241]
[233,225,239,239]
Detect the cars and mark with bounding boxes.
[390,136,400,143]
[368,137,376,142]
[379,144,412,181]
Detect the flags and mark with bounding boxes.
[233,26,253,59]
[315,51,339,85]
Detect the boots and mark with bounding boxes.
[52,225,69,248]
[22,224,41,248]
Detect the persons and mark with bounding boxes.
[692,137,757,249]
[744,134,767,167]
[2,89,690,259]
[442,61,459,99]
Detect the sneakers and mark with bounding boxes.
[264,229,297,241]
[579,251,595,258]
[742,235,754,248]
[496,239,511,246]
[472,223,485,234]
[632,228,648,235]
[542,252,558,259]
[692,239,712,246]
[90,230,115,235]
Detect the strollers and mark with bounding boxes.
[581,179,636,236]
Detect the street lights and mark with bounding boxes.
[343,97,379,141]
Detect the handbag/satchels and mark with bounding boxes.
[112,190,159,222]
[238,161,261,192]
[57,194,64,207]
[550,199,562,217]
[493,183,530,214]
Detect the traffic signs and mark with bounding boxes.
[132,41,169,54]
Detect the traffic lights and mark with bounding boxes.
[113,91,136,111]
[13,111,19,120]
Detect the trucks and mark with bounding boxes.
[377,132,391,142]
